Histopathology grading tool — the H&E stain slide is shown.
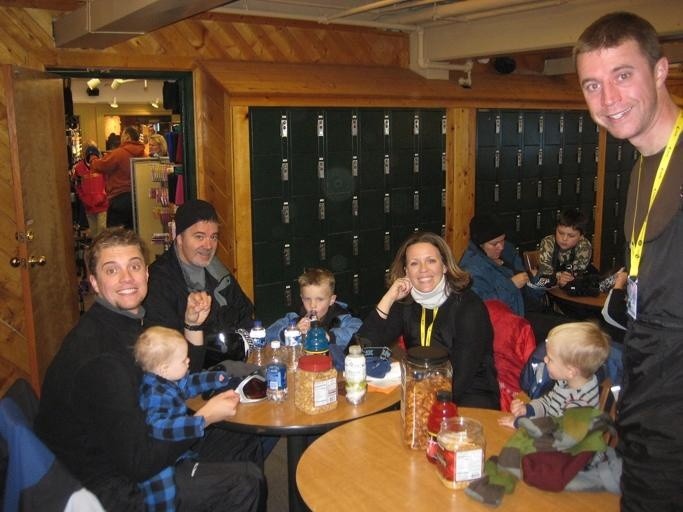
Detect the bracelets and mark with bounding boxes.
[184,323,207,330]
[376,306,390,317]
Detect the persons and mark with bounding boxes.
[91,126,148,231]
[354,231,501,412]
[458,212,572,341]
[529,208,592,289]
[148,133,168,158]
[597,266,639,409]
[571,8,682,511]
[73,137,109,243]
[142,197,258,371]
[262,265,364,371]
[33,226,268,512]
[132,324,232,511]
[497,321,610,430]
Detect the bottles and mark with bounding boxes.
[436,417,485,490]
[426,389,458,466]
[344,346,368,406]
[303,321,330,357]
[249,321,266,366]
[294,355,338,414]
[310,309,318,320]
[283,318,303,373]
[566,264,573,274]
[265,341,288,404]
[400,346,453,451]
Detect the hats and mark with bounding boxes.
[175,199,219,235]
[86,146,100,156]
[469,212,505,246]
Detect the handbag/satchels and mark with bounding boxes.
[204,331,245,369]
[566,271,601,297]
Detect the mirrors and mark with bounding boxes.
[104,114,173,157]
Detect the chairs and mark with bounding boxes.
[1,376,103,512]
[544,360,624,448]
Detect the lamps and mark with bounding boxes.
[111,79,148,92]
[87,78,101,90]
[150,97,166,109]
[110,97,119,109]
